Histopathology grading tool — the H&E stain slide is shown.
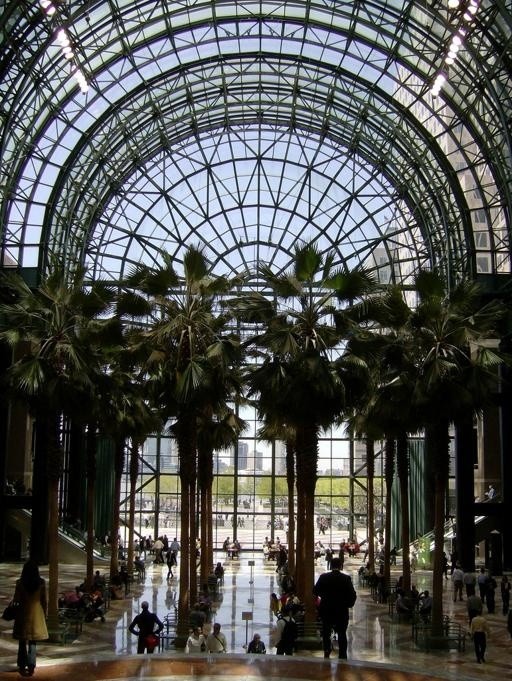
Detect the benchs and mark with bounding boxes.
[294,622,334,652]
[46,608,85,646]
[157,612,192,652]
[413,614,468,652]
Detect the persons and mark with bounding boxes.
[314,517,384,570]
[223,537,240,557]
[443,550,512,664]
[63,514,111,557]
[184,606,296,657]
[475,484,494,502]
[58,571,124,623]
[262,536,293,616]
[12,561,50,678]
[189,562,224,628]
[359,542,431,622]
[3,479,32,496]
[118,534,180,579]
[128,601,164,655]
[314,559,357,660]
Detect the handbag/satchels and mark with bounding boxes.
[144,634,160,648]
[2,597,19,620]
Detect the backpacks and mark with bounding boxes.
[279,617,298,648]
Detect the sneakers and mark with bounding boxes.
[17,665,34,674]
